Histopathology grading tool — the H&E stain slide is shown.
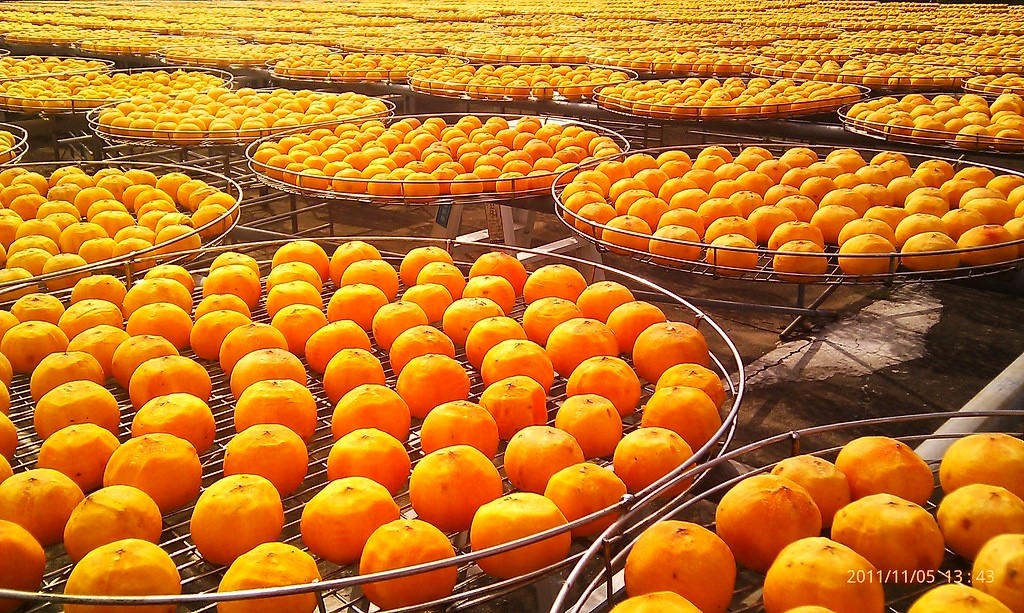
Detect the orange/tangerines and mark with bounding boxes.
[0,1,1023,612]
